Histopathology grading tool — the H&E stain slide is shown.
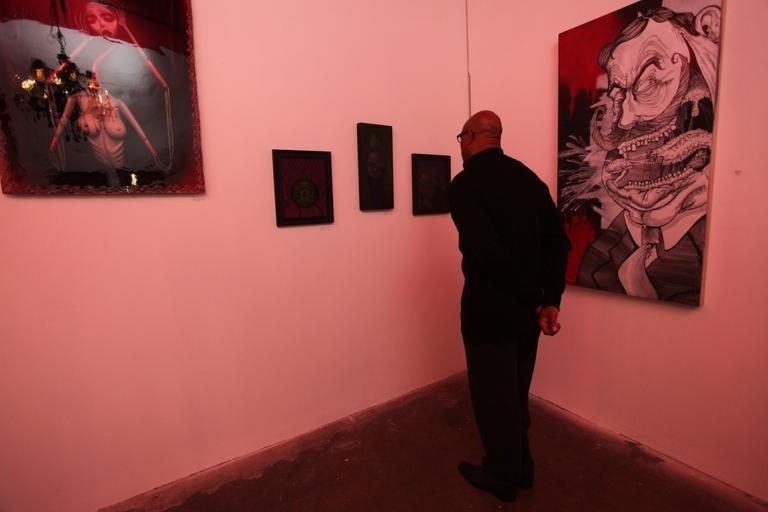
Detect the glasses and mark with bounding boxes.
[457,131,469,143]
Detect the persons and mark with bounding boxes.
[450,110,570,503]
[45,2,174,173]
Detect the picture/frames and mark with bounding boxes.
[272,150,334,227]
[412,154,451,215]
[357,123,394,210]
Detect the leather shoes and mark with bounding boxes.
[459,457,533,502]
